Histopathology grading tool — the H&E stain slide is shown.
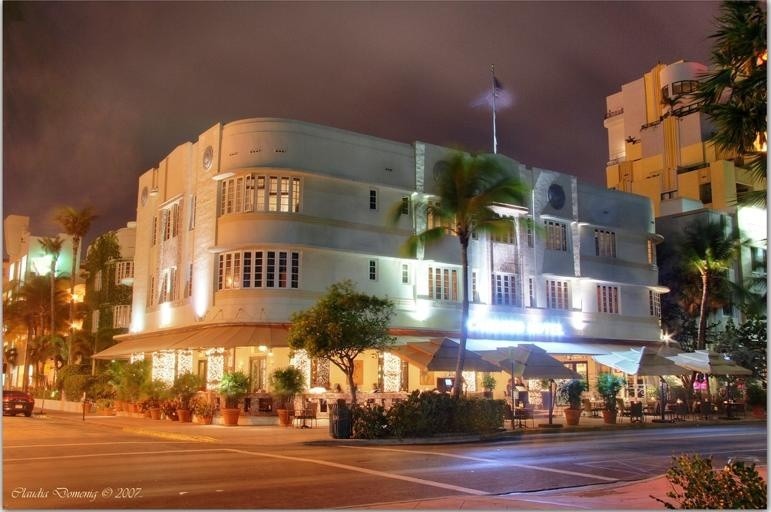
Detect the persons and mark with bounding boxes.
[308,383,381,394]
[507,377,525,407]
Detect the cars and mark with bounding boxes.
[3,391,35,416]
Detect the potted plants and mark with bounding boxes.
[483,374,497,399]
[268,362,306,429]
[560,378,587,425]
[84,360,250,425]
[596,370,626,423]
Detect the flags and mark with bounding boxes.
[494,77,505,100]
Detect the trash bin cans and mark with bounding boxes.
[328,403,356,439]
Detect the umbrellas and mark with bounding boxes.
[592,345,693,421]
[474,344,584,424]
[664,348,753,416]
[378,338,502,371]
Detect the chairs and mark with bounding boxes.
[583,398,744,424]
[505,403,535,429]
[286,402,319,428]
